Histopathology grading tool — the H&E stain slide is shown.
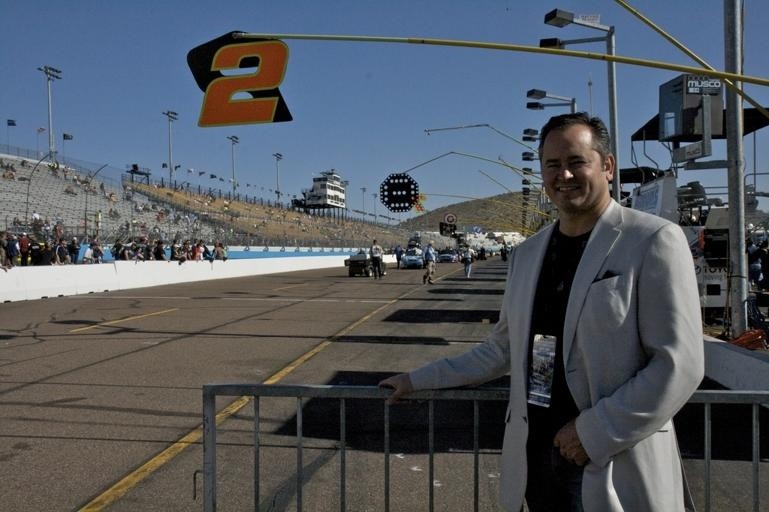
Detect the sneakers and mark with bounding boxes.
[423,278,434,285]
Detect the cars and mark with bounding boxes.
[401,240,514,268]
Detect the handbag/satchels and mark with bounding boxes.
[423,259,428,269]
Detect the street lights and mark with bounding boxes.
[163,110,178,188]
[272,153,283,206]
[361,188,378,225]
[521,8,622,239]
[226,135,239,199]
[37,65,62,161]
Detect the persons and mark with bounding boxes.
[266,208,514,284]
[2,160,228,265]
[378,111,705,512]
